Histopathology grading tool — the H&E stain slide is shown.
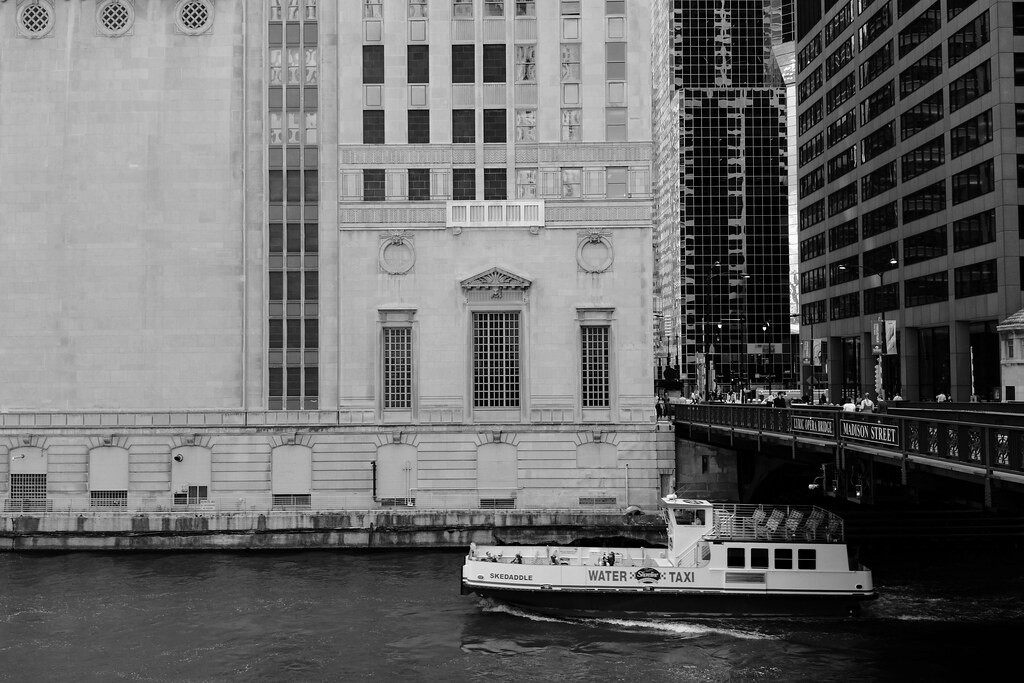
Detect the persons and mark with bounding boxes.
[678,394,687,404]
[607,552,615,566]
[690,390,701,405]
[843,392,888,414]
[514,555,523,564]
[481,551,502,563]
[551,550,559,565]
[936,391,953,402]
[821,394,826,403]
[758,391,787,408]
[654,392,670,419]
[691,514,701,525]
[893,393,903,400]
[729,389,736,403]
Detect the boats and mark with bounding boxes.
[460,485,879,616]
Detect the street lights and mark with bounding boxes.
[705,261,751,401]
[838,258,897,400]
[706,323,722,401]
[760,320,772,395]
